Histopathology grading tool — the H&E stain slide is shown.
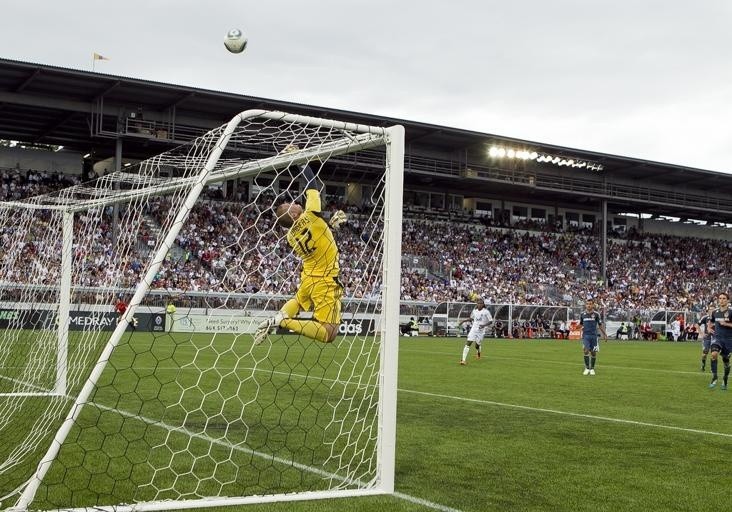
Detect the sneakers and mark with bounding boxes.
[258,310,290,329]
[254,320,268,346]
[477,353,481,358]
[708,378,718,388]
[721,380,728,389]
[590,369,595,375]
[583,368,589,376]
[460,360,467,365]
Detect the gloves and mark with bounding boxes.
[328,209,347,232]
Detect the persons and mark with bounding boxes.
[570,299,608,376]
[703,292,732,391]
[458,298,494,365]
[1,158,732,338]
[693,305,717,372]
[252,142,347,347]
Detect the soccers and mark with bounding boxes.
[223,28,248,52]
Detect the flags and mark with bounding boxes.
[94,53,109,62]
